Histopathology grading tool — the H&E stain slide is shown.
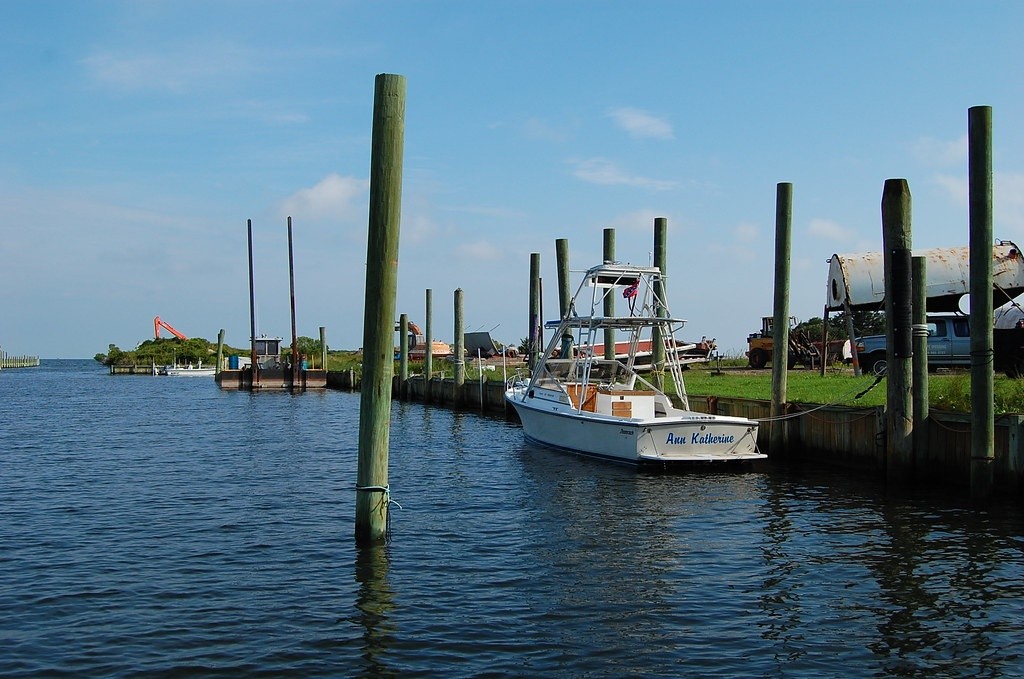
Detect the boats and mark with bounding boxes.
[165,358,216,377]
[539,337,718,371]
[215,216,328,393]
[500,251,768,465]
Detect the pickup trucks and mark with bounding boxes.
[852,315,971,378]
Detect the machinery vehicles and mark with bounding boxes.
[745,316,821,370]
[394,321,452,360]
